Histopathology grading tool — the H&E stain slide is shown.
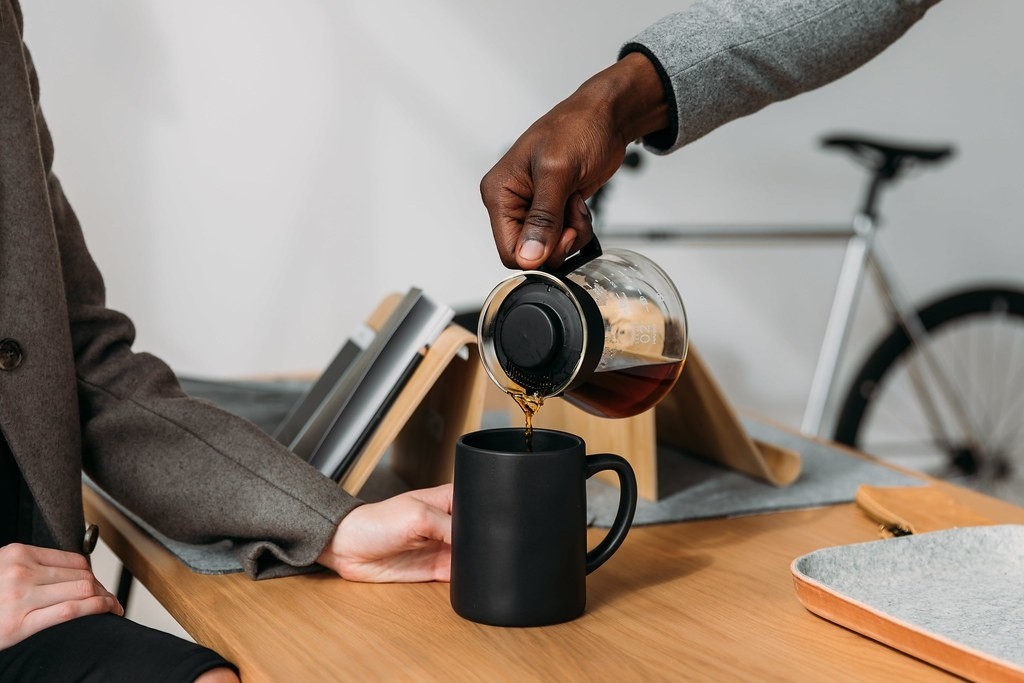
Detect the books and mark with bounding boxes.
[293,286,457,488]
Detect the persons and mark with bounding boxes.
[480,0,941,271]
[0,0,454,683]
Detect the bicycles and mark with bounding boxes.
[575,130,1023,510]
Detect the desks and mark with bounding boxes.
[82,374,1024,683]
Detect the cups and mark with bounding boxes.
[450,427,638,628]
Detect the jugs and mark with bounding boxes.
[477,233,689,419]
